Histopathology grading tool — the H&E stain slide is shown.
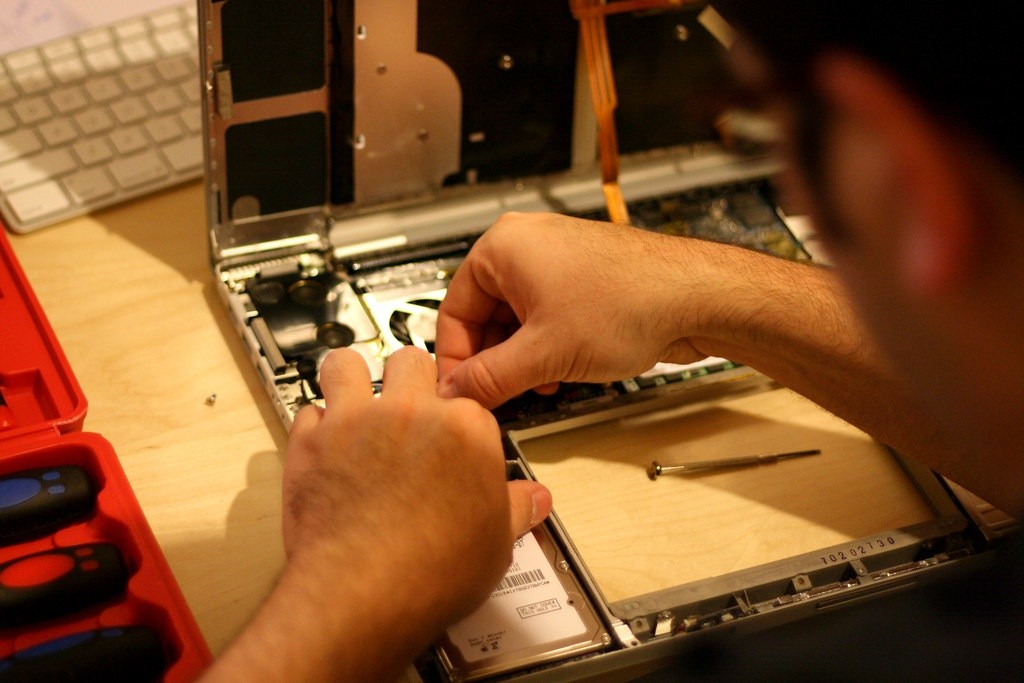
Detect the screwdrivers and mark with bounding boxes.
[650,448,821,479]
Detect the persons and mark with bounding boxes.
[198,0,1024,683]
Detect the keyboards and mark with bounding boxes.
[0,6,206,234]
